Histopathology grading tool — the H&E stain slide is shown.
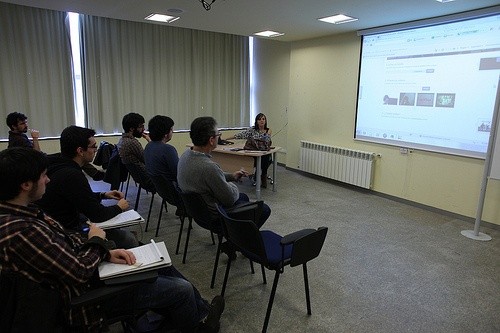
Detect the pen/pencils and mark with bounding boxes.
[86,221,108,241]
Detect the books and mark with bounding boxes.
[89,207,144,235]
[229,148,267,154]
[96,238,172,276]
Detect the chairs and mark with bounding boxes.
[93,141,317,333]
[0,268,122,333]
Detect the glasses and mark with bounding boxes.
[206,132,222,139]
[136,125,144,129]
[76,143,98,151]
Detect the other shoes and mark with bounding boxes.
[221,241,237,260]
[176,209,190,217]
[253,172,257,180]
[200,296,225,333]
[92,171,106,181]
[262,179,267,188]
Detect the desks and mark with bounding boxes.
[104,271,159,285]
[186,144,281,199]
[290,226,328,267]
[82,199,141,233]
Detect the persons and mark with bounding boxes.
[0,146,224,333]
[117,110,152,183]
[34,123,141,250]
[5,112,107,181]
[224,112,273,188]
[177,117,271,261]
[140,115,191,216]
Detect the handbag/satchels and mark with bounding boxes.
[244,134,270,151]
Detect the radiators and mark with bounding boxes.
[299,140,382,190]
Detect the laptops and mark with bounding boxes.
[217,136,235,145]
